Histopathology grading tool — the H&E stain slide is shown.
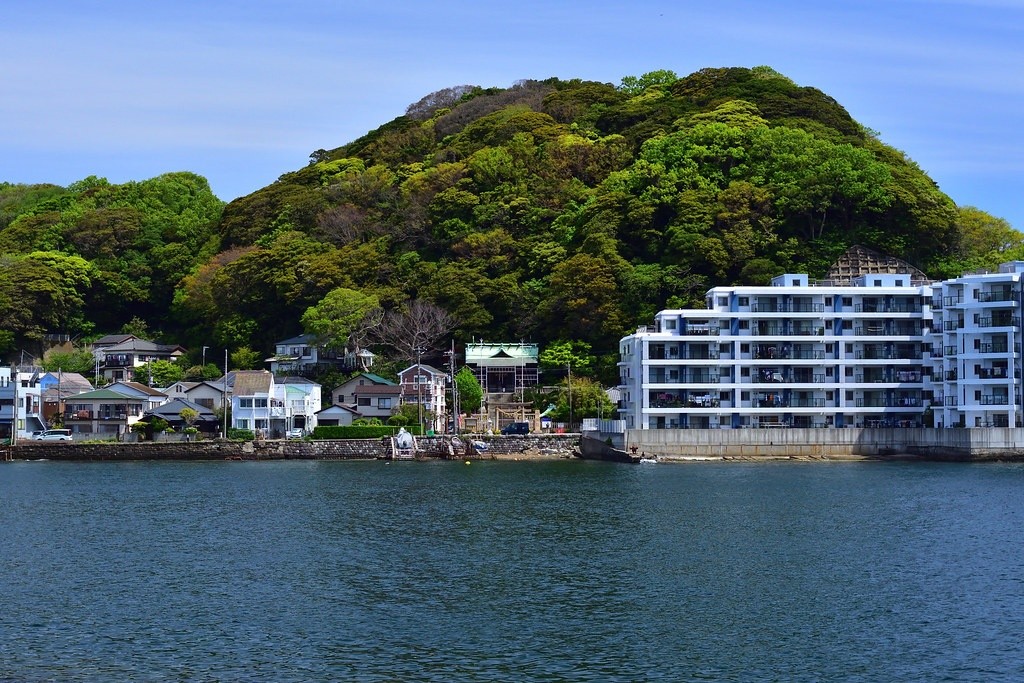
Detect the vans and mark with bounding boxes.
[501,422,530,436]
[32,431,43,439]
[35,429,73,440]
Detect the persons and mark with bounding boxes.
[630,442,638,454]
[255,426,263,439]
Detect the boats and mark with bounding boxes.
[452,437,463,447]
[397,429,412,449]
[472,440,489,452]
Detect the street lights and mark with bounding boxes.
[203,346,209,366]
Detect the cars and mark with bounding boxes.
[290,428,305,438]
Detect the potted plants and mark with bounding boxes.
[185,427,197,442]
